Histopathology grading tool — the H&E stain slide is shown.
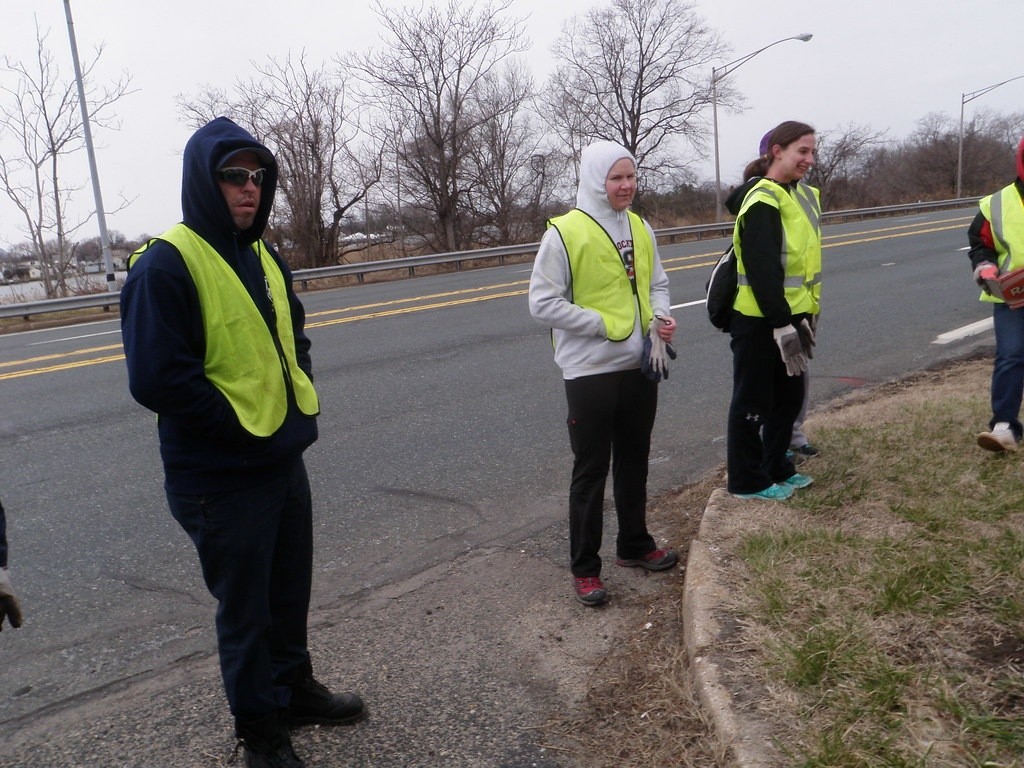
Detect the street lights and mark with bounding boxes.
[711,31,813,235]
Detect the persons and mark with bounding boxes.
[968,139,1024,452]
[119,117,366,768]
[0,500,22,632]
[529,142,678,605]
[723,121,823,498]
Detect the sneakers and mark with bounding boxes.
[573,576,606,605]
[797,445,821,458]
[617,548,677,571]
[786,473,814,488]
[976,420,1020,453]
[787,451,805,466]
[734,483,794,501]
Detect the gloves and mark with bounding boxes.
[794,319,817,360]
[973,262,999,296]
[775,323,809,376]
[0,567,24,633]
[642,317,678,383]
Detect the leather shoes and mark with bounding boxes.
[235,718,303,768]
[297,676,367,724]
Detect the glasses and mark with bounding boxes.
[219,167,268,191]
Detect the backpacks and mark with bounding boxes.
[703,190,778,333]
